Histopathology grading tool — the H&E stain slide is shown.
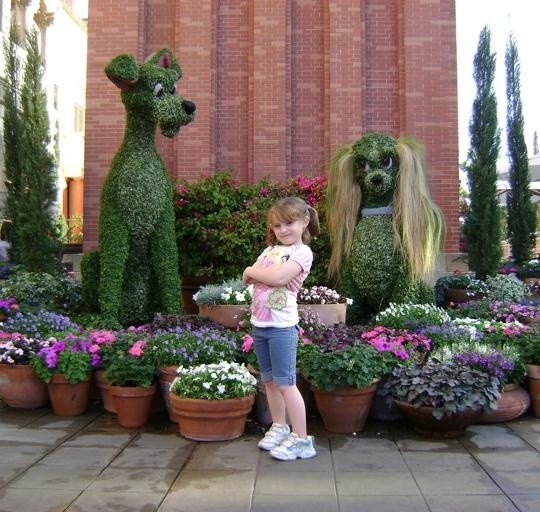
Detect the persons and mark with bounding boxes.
[0,233,12,262]
[242,196,322,463]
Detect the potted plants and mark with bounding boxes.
[377,362,501,440]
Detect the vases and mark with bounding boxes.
[482,384,532,424]
[525,363,540,418]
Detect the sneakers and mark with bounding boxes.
[258,423,291,451]
[271,433,316,460]
